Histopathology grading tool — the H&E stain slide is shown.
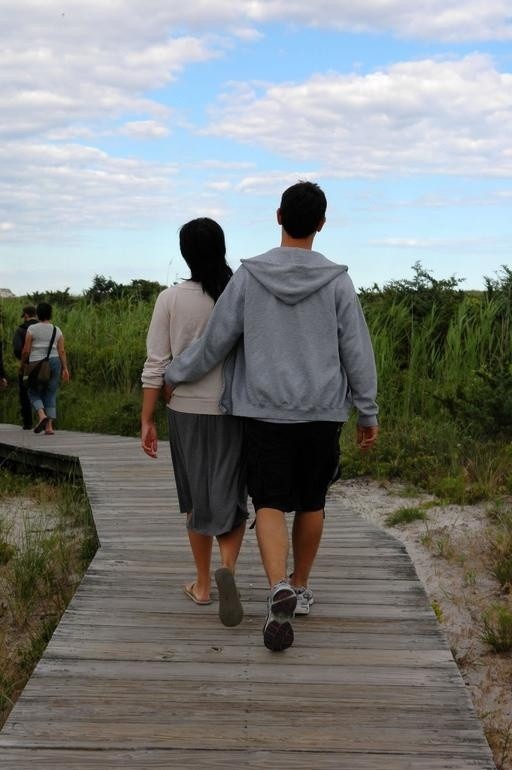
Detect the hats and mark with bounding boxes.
[21,306,35,318]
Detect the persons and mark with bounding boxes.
[141,217,251,628]
[0,339,8,389]
[21,304,69,435]
[163,179,378,649]
[13,306,39,429]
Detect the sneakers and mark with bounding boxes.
[23,426,32,429]
[263,581,297,650]
[292,587,314,613]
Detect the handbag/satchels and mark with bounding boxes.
[23,360,50,381]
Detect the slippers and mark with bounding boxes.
[215,568,243,627]
[34,416,54,434]
[184,581,213,604]
